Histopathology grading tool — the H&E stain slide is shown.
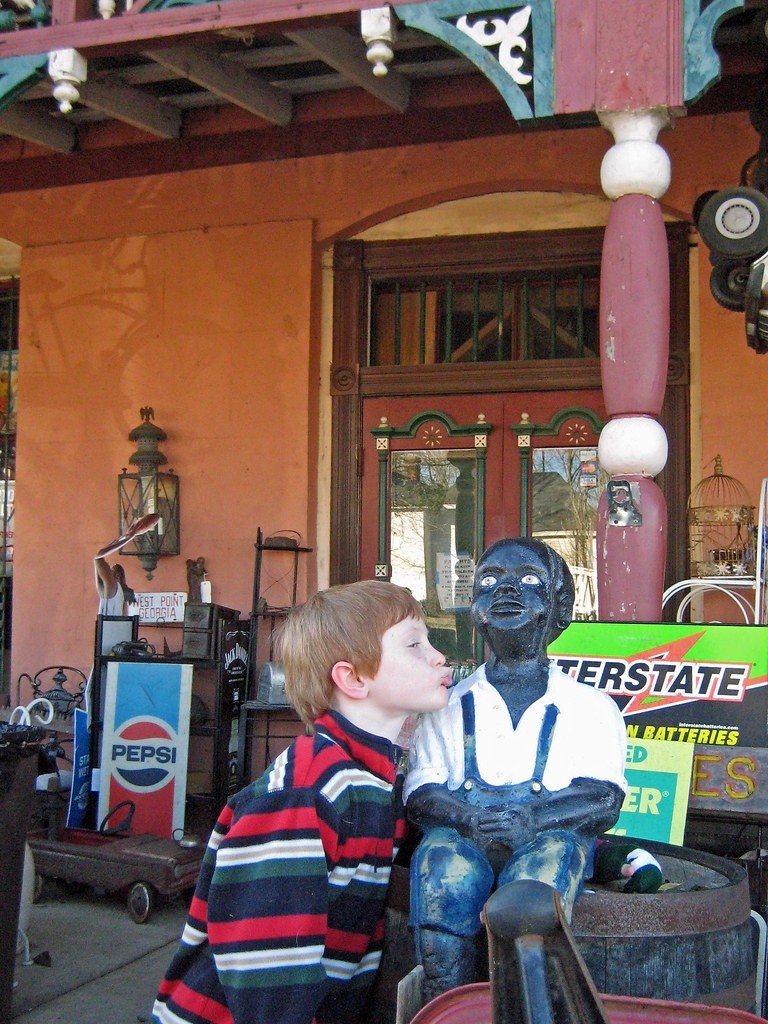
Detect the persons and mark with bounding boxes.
[85,520,146,736]
[400,533,632,1009]
[147,578,666,1024]
